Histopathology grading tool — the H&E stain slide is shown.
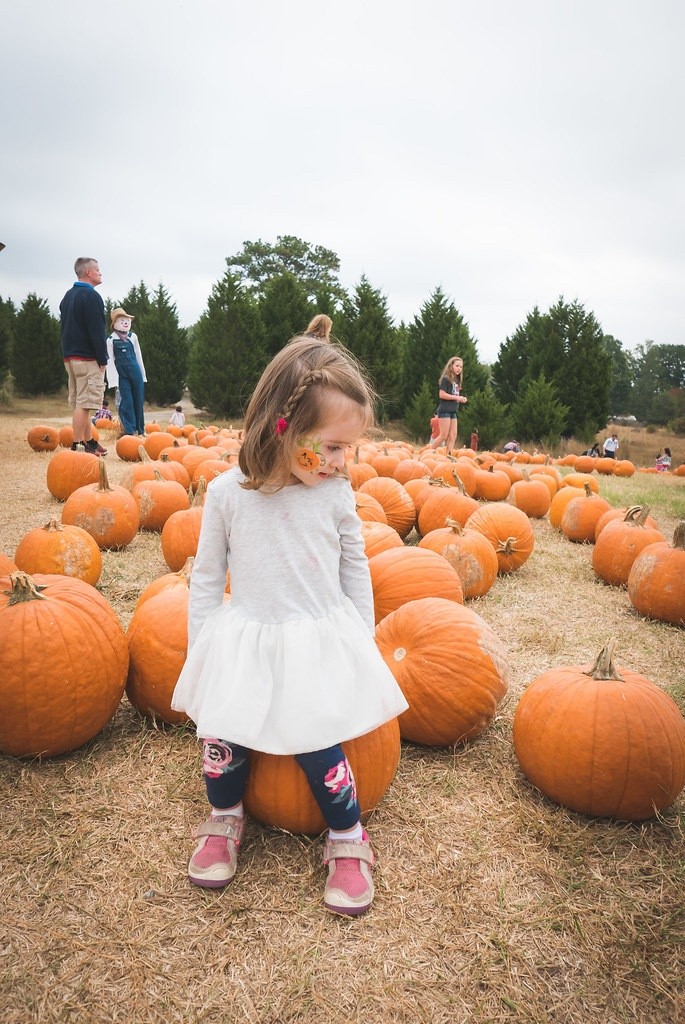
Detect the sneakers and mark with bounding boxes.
[186,812,247,888]
[72,442,102,458]
[88,439,107,455]
[324,829,376,916]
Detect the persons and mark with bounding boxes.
[171,340,409,916]
[587,434,619,459]
[106,308,147,437]
[658,448,671,471]
[430,412,444,446]
[471,428,478,451]
[304,315,332,341]
[169,406,185,428]
[504,440,520,453]
[431,358,467,457]
[59,257,108,457]
[92,401,113,425]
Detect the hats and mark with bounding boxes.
[109,308,135,329]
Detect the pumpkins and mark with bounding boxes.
[0,418,685,837]
